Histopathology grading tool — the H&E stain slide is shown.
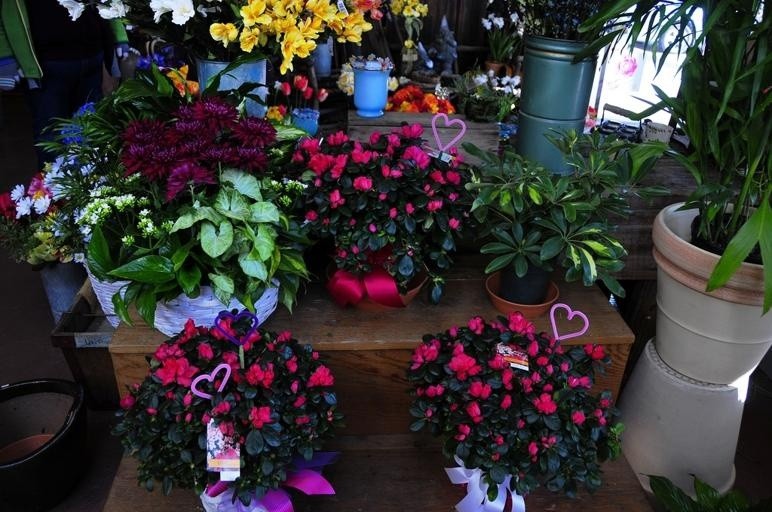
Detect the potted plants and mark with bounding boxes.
[575,0,772,386]
[466,132,635,320]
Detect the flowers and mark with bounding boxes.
[363,1,429,56]
[209,0,371,73]
[116,315,341,500]
[336,63,355,99]
[62,0,270,62]
[6,148,112,261]
[480,1,600,39]
[386,84,453,115]
[102,79,302,273]
[296,125,461,295]
[463,73,519,116]
[403,316,622,501]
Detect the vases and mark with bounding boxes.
[196,58,266,118]
[314,45,334,81]
[517,34,594,174]
[325,258,429,314]
[150,282,281,338]
[37,258,82,324]
[464,98,491,123]
[349,54,397,118]
[81,258,134,326]
